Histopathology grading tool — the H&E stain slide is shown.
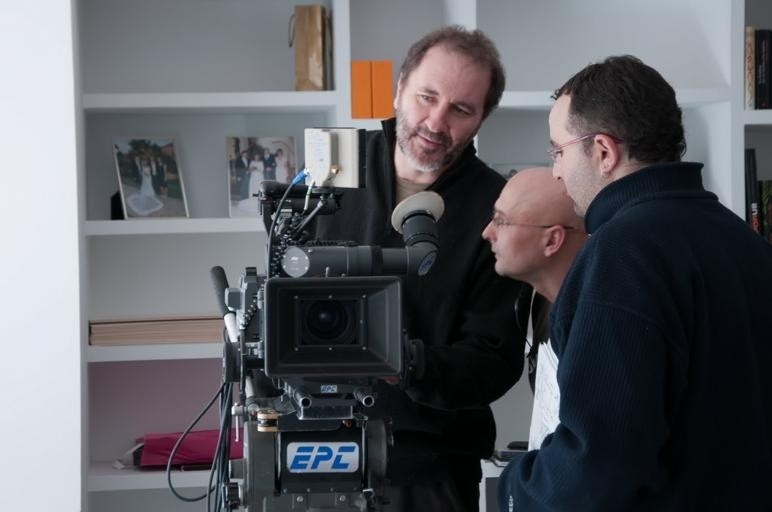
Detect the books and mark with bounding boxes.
[745,25,772,249]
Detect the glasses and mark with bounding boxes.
[547,133,624,162]
[492,216,575,229]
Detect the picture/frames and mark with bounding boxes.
[105,133,300,219]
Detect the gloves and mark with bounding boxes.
[367,329,426,397]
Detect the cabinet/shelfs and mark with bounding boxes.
[68,1,772,507]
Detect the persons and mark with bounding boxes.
[282,24,533,511]
[481,167,594,455]
[497,52,772,512]
[236,139,290,216]
[128,149,168,216]
